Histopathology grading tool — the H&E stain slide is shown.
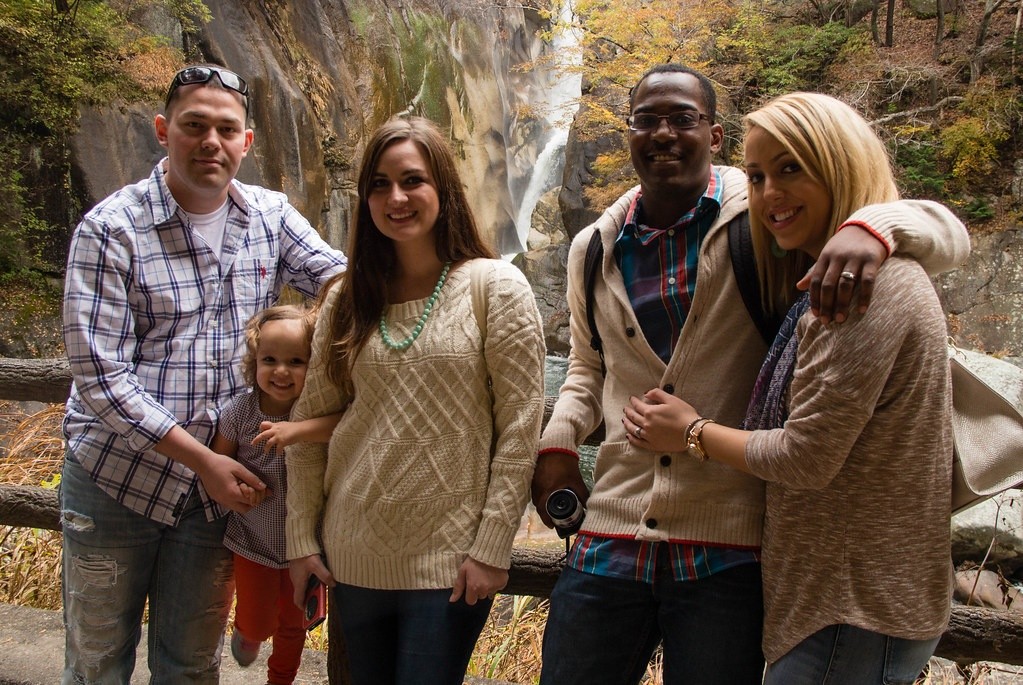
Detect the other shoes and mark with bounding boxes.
[231,628,260,666]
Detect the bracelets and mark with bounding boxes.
[684,417,709,444]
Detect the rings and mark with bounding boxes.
[635,427,641,437]
[841,272,855,280]
[488,594,494,599]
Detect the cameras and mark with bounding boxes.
[546,487,587,538]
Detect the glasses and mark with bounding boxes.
[626,112,714,131]
[165,66,249,119]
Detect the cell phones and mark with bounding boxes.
[303,573,326,631]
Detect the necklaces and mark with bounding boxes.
[379,257,451,351]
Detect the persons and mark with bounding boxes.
[622,90,955,685]
[207,303,340,685]
[530,63,969,685]
[285,118,546,685]
[60,64,349,685]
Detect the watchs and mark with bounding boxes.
[686,419,717,461]
